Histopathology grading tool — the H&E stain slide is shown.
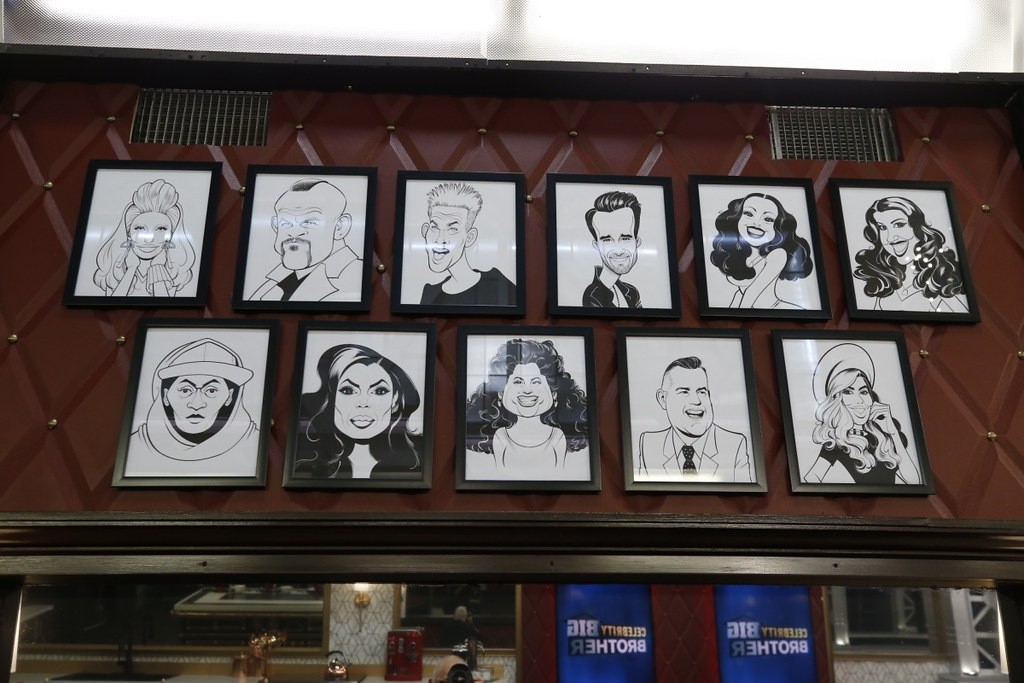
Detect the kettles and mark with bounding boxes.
[325,651,353,683]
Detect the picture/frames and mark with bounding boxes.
[611,324,769,495]
[389,163,529,320]
[230,162,381,313]
[687,172,834,325]
[452,321,606,497]
[543,169,684,322]
[278,315,437,492]
[825,174,983,327]
[109,314,284,494]
[766,325,935,497]
[61,156,225,310]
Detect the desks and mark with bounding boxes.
[171,587,326,648]
